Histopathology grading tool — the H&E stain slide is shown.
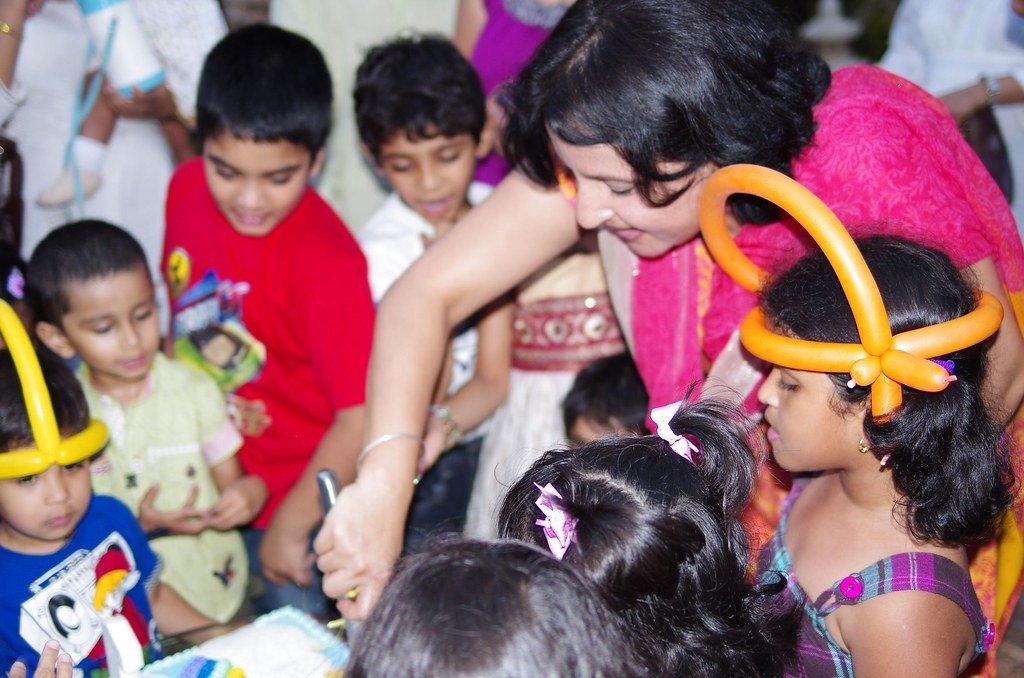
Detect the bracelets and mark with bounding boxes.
[356,434,426,475]
[157,113,177,122]
[1,23,22,41]
[431,405,462,449]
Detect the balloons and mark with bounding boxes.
[699,164,1004,422]
[0,297,109,479]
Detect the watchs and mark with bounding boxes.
[981,77,999,107]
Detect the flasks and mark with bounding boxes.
[77,0,166,100]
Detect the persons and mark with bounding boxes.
[879,0,1024,241]
[496,402,809,678]
[315,0,1024,641]
[755,235,1024,678]
[0,341,257,678]
[159,23,374,614]
[0,0,454,338]
[341,541,644,678]
[452,0,579,186]
[351,34,654,540]
[24,219,268,623]
